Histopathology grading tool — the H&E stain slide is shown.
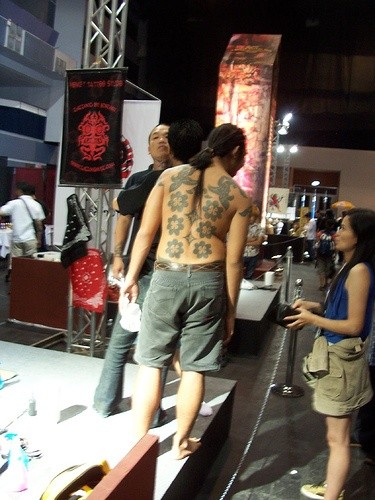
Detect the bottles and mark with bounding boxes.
[8,435,30,492]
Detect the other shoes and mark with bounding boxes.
[319,287,325,291]
[198,401,213,417]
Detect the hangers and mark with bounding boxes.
[0,222,13,230]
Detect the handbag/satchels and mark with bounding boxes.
[301,352,318,389]
[70,248,107,313]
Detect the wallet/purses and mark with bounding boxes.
[268,303,304,330]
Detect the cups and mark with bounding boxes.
[264,271,275,286]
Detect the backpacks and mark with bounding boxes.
[318,238,332,256]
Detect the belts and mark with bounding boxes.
[155,262,225,272]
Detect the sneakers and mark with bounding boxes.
[300,480,345,500]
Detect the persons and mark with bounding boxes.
[0,180,52,271]
[282,208,374,500]
[93,120,212,427]
[119,124,253,460]
